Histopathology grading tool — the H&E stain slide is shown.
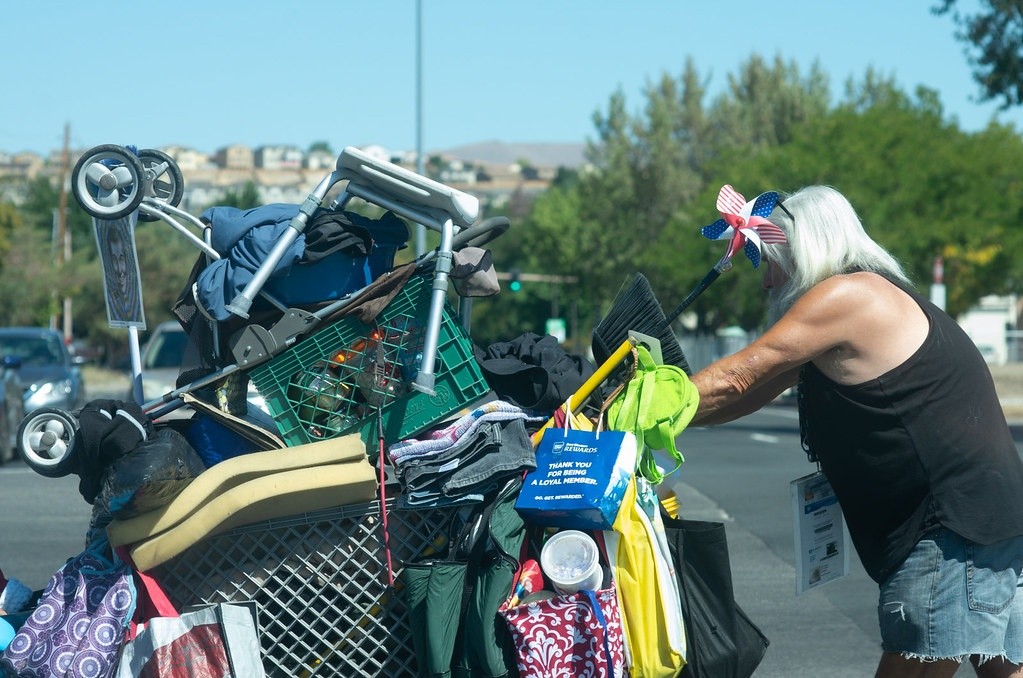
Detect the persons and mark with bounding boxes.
[682,185,1023,678]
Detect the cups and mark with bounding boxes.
[552,564,604,596]
[541,530,599,594]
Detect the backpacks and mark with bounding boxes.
[607,345,699,484]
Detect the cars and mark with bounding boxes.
[0,327,88,443]
[127,319,273,416]
[0,355,25,464]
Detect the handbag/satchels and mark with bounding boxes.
[512,395,637,531]
[0,534,137,678]
[115,570,265,678]
[656,497,770,678]
[499,526,629,678]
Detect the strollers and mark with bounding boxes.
[18,144,480,502]
